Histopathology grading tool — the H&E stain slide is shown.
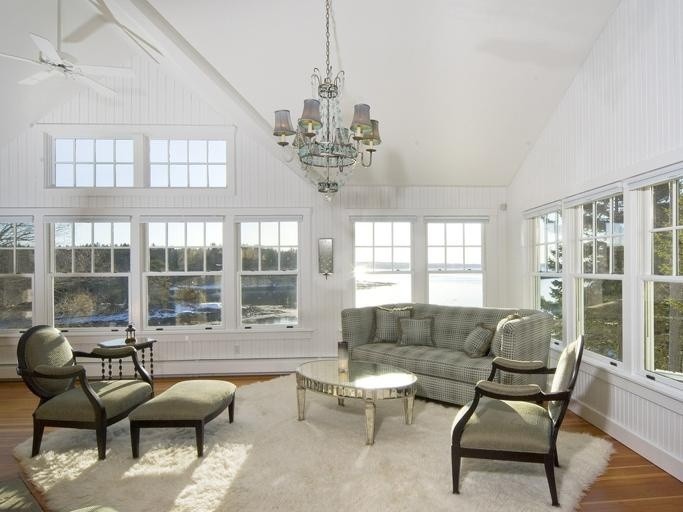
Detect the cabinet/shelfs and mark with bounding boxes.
[93,333,157,378]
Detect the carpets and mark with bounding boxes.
[9,366,616,509]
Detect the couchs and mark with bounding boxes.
[340,302,549,405]
[128,378,237,456]
[447,339,586,504]
[14,322,149,460]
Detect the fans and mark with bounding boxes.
[0,1,133,98]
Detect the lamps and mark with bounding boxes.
[317,236,338,284]
[270,0,382,196]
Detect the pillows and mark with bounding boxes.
[461,322,497,359]
[372,305,413,342]
[491,313,521,357]
[400,317,436,348]
